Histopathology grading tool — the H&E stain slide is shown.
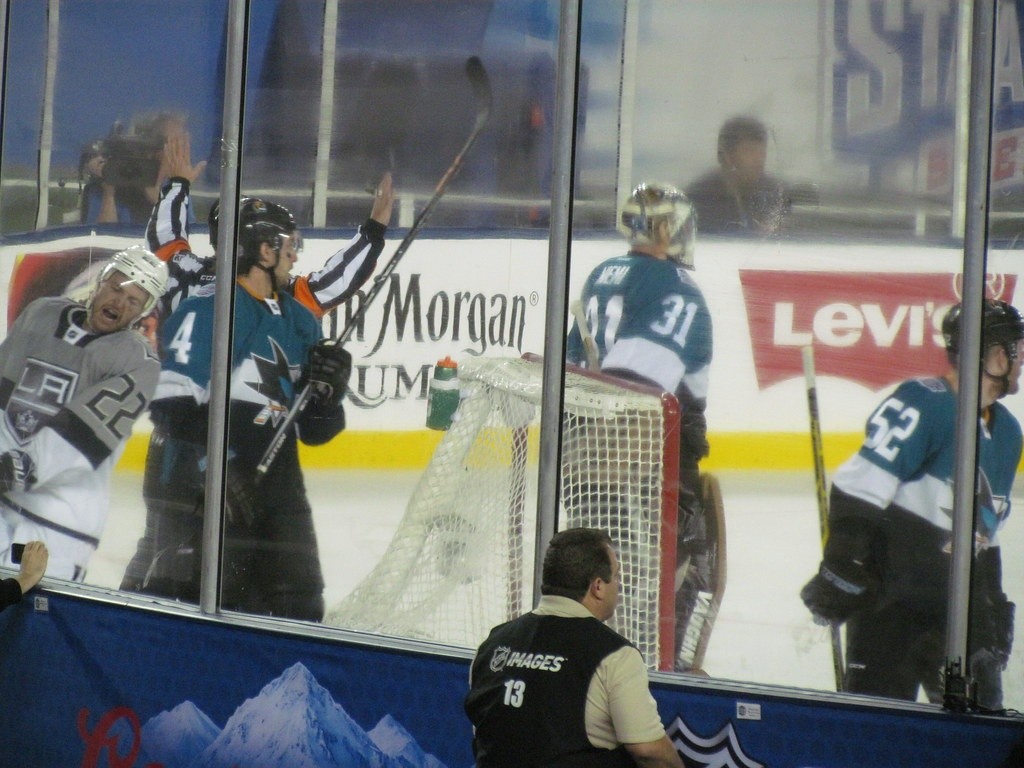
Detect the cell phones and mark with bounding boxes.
[11,543,26,564]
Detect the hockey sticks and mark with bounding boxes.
[799,342,848,694]
[569,297,692,603]
[246,51,498,489]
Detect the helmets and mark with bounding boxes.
[101,245,169,318]
[208,193,249,245]
[615,181,695,245]
[239,201,297,251]
[942,298,1024,360]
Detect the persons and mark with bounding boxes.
[142,199,352,622]
[0,245,169,583]
[464,528,685,768]
[691,116,784,240]
[564,181,728,675]
[118,130,393,592]
[0,541,48,612]
[801,298,1024,711]
[83,108,197,226]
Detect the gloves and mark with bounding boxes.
[0,449,37,492]
[987,601,1015,672]
[800,558,879,629]
[183,441,252,524]
[299,346,352,409]
